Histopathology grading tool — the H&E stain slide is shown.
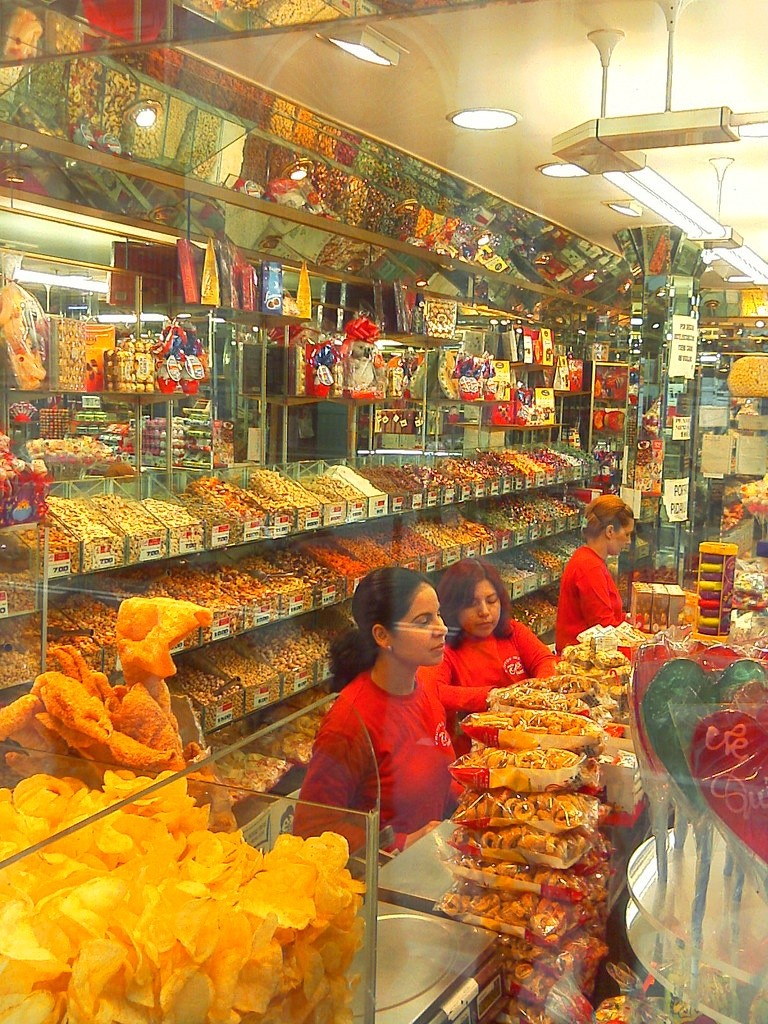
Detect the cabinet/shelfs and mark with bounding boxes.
[1,120,600,792]
[0,187,593,500]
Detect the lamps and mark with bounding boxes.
[552,107,767,285]
[314,23,411,67]
[123,99,164,126]
[282,160,313,181]
[608,202,642,217]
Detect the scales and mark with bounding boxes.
[339,901,508,1024]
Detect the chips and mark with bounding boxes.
[0,772,367,1024]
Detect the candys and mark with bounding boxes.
[1,47,473,255]
[0,440,588,736]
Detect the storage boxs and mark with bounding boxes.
[232,786,302,853]
[170,279,184,303]
[106,271,136,305]
[111,241,148,271]
[143,275,170,305]
[260,261,284,313]
[631,582,686,634]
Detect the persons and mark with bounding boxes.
[291,557,562,852]
[555,495,635,656]
